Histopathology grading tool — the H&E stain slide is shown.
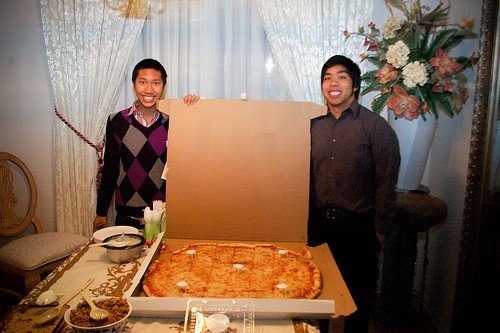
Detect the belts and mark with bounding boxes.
[321,206,355,225]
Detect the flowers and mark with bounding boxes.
[342,1,479,123]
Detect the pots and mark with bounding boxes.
[101,234,146,264]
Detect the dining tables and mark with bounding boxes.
[0,236,321,333]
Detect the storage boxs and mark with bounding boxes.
[119,92,358,319]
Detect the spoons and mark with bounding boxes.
[78,288,108,320]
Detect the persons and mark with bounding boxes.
[93,58,200,233]
[311,55,400,333]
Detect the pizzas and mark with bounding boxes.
[142,243,321,300]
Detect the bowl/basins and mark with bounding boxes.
[92,226,138,244]
[64,296,132,333]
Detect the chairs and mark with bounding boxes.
[0,151,90,300]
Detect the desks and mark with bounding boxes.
[374,190,447,324]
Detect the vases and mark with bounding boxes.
[387,107,438,190]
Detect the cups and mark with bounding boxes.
[143,221,160,240]
